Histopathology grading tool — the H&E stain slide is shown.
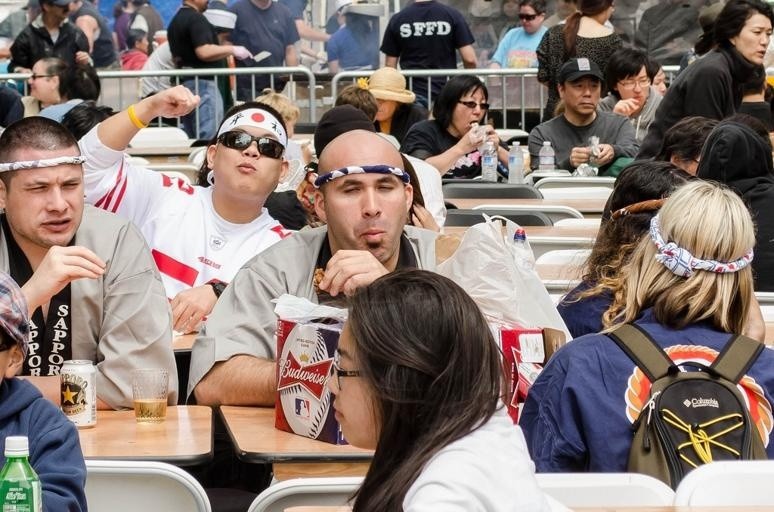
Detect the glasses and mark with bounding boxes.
[328,360,365,391]
[219,130,285,160]
[458,100,490,111]
[618,77,652,89]
[517,12,540,22]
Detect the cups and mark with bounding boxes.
[129,368,169,422]
[765,71,774,86]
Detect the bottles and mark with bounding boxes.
[507,142,526,185]
[0,430,40,511]
[539,142,554,175]
[512,227,525,248]
[481,140,498,180]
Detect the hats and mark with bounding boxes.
[357,67,416,104]
[201,8,239,31]
[557,57,605,83]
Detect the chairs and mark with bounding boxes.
[49,121,773,512]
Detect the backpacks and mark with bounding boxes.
[603,321,770,495]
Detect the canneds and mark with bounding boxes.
[60,359,98,429]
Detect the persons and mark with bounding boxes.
[180,128,506,408]
[0,114,183,410]
[0,263,88,512]
[324,266,571,512]
[513,176,772,494]
